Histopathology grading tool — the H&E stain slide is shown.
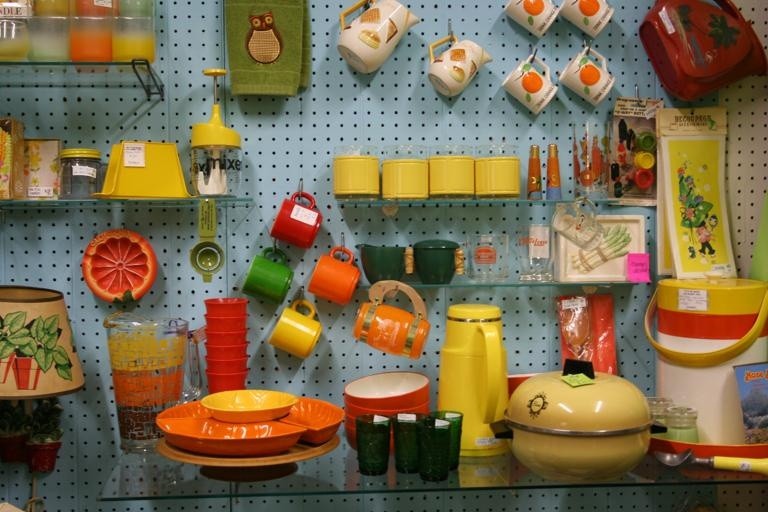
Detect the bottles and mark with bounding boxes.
[525,144,545,201]
[56,148,104,200]
[545,143,563,200]
[189,102,242,198]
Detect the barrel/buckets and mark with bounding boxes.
[643,274,764,444]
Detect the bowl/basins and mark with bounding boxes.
[344,369,431,456]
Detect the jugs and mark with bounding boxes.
[438,303,510,458]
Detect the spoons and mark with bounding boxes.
[653,447,768,477]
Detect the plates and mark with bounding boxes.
[155,388,346,469]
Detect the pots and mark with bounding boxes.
[491,359,670,481]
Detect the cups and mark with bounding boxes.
[354,408,465,482]
[352,280,430,360]
[411,239,460,285]
[467,233,509,282]
[550,196,604,251]
[336,0,419,74]
[559,46,615,107]
[265,299,323,358]
[558,1,616,39]
[268,192,323,251]
[380,159,430,199]
[360,245,408,286]
[330,155,382,198]
[646,398,674,440]
[514,223,554,281]
[102,314,203,454]
[572,118,612,199]
[240,248,293,304]
[0,0,158,65]
[666,405,700,445]
[307,246,360,306]
[504,1,560,38]
[204,294,250,394]
[426,34,493,99]
[473,155,522,199]
[428,155,475,199]
[500,54,558,116]
